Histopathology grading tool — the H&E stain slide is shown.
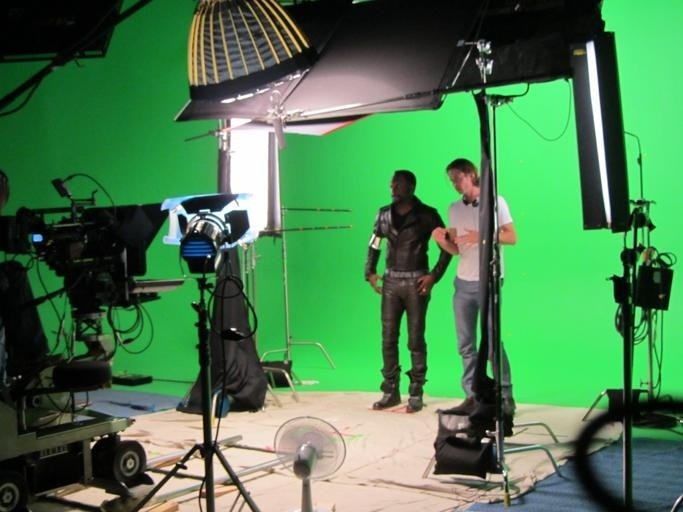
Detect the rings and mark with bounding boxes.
[422,288,426,292]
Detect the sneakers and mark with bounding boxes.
[451,398,479,413]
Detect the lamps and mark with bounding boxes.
[215,117,281,346]
[169,0,317,112]
[573,30,652,510]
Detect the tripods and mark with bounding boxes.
[421,237,572,508]
[581,153,683,425]
[450,239,559,445]
[210,208,336,420]
[131,275,260,512]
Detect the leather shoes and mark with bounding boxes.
[406,394,424,413]
[372,389,402,409]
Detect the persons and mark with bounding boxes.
[364,169,453,414]
[429,158,518,436]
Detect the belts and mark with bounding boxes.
[383,266,428,280]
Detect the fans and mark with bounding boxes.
[273,415,346,512]
